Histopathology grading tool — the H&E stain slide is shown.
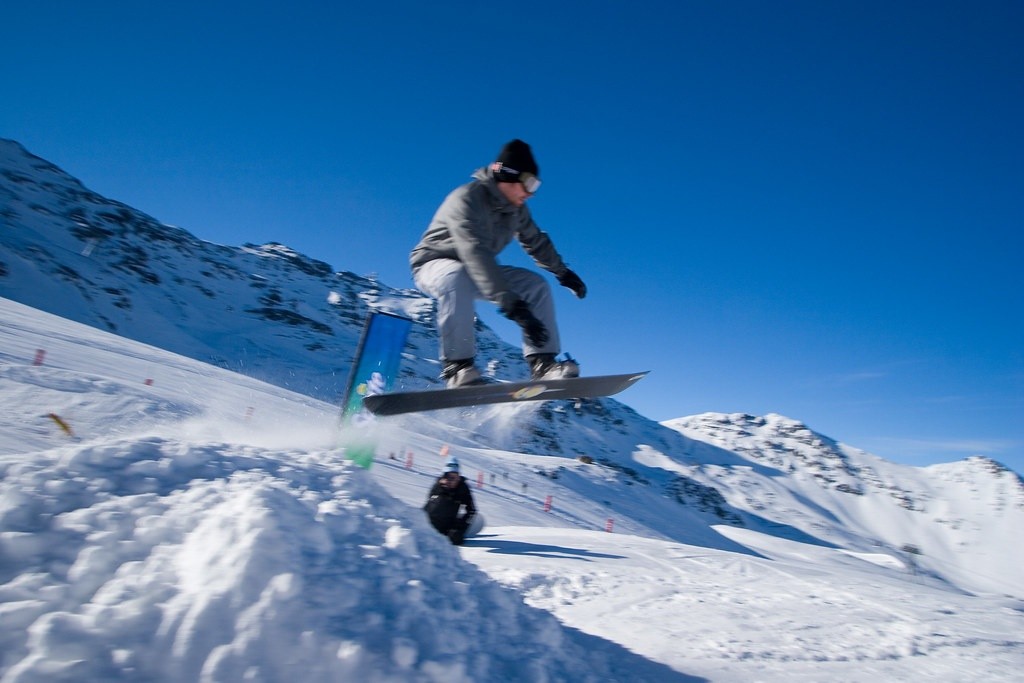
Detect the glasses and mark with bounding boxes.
[445,476,458,481]
[518,173,544,193]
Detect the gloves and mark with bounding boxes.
[557,269,589,299]
[505,301,551,347]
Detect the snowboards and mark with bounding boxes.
[361,371,651,417]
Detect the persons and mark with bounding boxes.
[424,457,476,545]
[409,138,586,388]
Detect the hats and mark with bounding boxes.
[492,139,539,183]
[444,457,461,473]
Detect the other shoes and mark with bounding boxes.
[442,359,483,389]
[527,358,579,380]
